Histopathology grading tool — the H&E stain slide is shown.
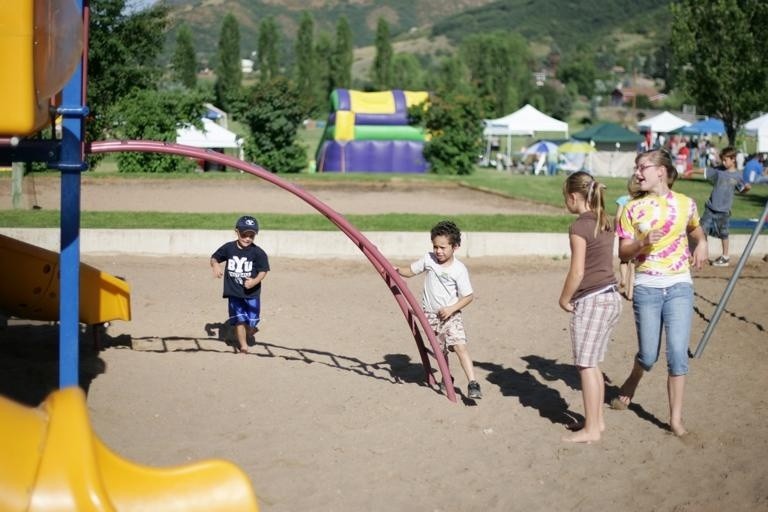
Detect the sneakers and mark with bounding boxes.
[440,377,454,392]
[711,256,730,266]
[468,380,482,399]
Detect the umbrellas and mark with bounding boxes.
[526,140,558,154]
[556,139,598,157]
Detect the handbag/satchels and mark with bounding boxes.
[617,262,635,301]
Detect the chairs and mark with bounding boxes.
[497,152,588,176]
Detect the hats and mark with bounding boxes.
[236,216,258,235]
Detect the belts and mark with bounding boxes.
[601,286,618,294]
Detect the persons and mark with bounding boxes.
[558,171,621,442]
[638,131,768,185]
[616,148,709,436]
[210,216,271,355]
[381,221,484,399]
[684,147,753,267]
